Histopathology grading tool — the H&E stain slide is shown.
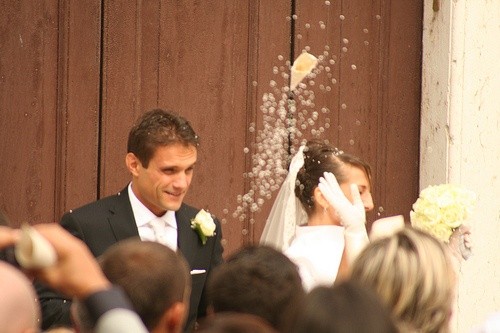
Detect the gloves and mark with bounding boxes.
[318,171,367,241]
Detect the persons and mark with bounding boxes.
[33,110,224,333]
[0,223,455,333]
[286,138,472,293]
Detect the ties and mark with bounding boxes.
[148,221,168,247]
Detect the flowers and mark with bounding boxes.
[410,184,477,260]
[190,209,217,244]
